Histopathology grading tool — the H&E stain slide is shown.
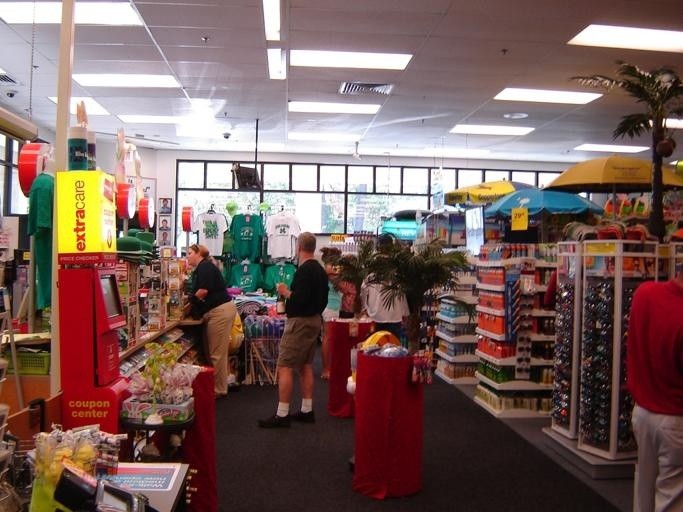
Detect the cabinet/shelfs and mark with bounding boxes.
[57,252,201,512]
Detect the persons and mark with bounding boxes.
[359,270,410,341]
[181,244,237,399]
[160,199,171,212]
[159,218,170,230]
[256,230,327,428]
[627,270,682,511]
[160,232,171,245]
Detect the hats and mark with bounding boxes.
[136,232,155,244]
[116,236,146,265]
[140,241,152,260]
[128,228,143,237]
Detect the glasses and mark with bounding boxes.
[520,314,531,321]
[579,281,613,445]
[519,294,533,300]
[516,368,530,372]
[519,304,532,310]
[618,287,634,440]
[517,347,530,352]
[517,358,529,363]
[518,337,530,342]
[519,324,532,331]
[550,283,573,424]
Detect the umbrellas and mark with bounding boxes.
[541,155,683,219]
[445,178,540,203]
[484,188,604,242]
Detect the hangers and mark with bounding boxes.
[276,205,287,216]
[206,204,216,215]
[275,258,286,266]
[243,205,253,215]
[241,256,251,265]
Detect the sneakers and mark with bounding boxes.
[259,414,290,428]
[289,408,314,422]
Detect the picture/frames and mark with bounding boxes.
[158,215,171,230]
[158,198,172,214]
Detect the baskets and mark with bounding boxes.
[11,438,119,488]
[6,352,51,375]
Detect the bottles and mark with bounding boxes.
[442,283,474,297]
[476,290,551,312]
[477,268,555,286]
[437,359,474,379]
[478,244,556,262]
[437,321,476,338]
[478,357,554,385]
[477,384,554,411]
[476,334,556,360]
[443,265,476,278]
[437,298,469,319]
[475,312,555,335]
[438,339,475,357]
